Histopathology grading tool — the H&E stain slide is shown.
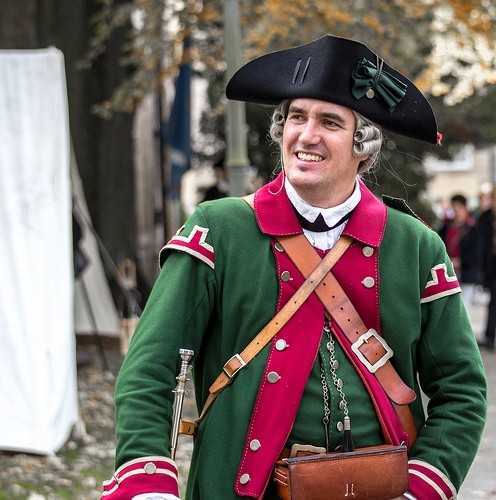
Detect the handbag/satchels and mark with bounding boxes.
[273,445,409,500]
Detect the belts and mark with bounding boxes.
[271,444,336,469]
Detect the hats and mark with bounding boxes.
[226,35,444,146]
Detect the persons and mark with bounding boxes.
[179,151,230,218]
[97,35,489,500]
[430,183,495,351]
